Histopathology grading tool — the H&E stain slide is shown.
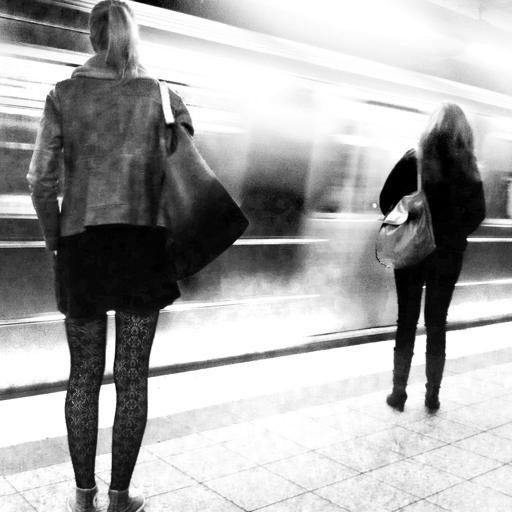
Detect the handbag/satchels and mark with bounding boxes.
[159,121,249,281]
[374,191,437,270]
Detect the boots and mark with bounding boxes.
[386,347,413,411]
[425,351,446,413]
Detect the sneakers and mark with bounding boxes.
[107,487,146,512]
[67,482,99,512]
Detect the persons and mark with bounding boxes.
[25,0,192,512]
[380,102,488,413]
